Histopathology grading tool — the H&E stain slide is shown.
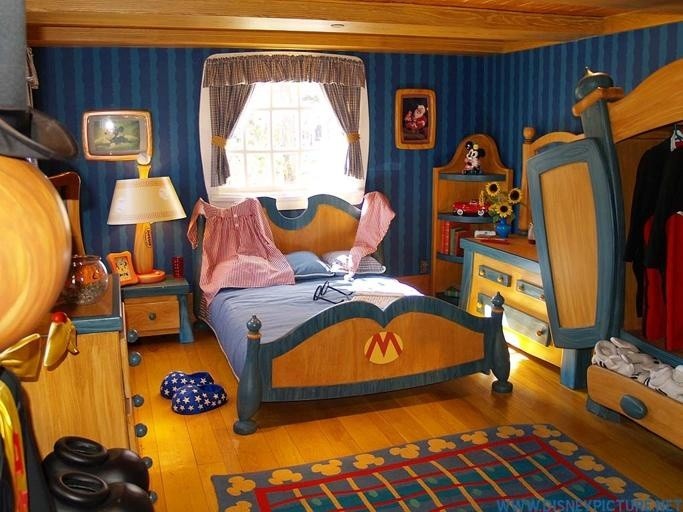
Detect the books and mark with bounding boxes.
[440,220,475,258]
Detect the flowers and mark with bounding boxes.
[478,180,520,216]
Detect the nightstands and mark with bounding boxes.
[121,274,195,345]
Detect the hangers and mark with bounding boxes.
[672,124,683,141]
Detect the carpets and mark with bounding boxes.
[210,422,681,512]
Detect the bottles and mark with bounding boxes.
[527,223,536,245]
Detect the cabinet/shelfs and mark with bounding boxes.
[430,133,511,304]
[524,52,683,453]
[17,268,157,502]
[456,230,587,389]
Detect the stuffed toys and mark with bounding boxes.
[462,139,486,176]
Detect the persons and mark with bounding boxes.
[405,102,428,136]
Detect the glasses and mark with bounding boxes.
[313,280,354,304]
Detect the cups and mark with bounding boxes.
[173,255,184,279]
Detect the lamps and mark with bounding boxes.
[105,154,183,279]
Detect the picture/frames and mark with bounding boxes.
[105,251,139,287]
[393,88,435,150]
[83,110,154,160]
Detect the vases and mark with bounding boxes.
[496,217,511,238]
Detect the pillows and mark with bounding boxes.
[321,244,386,279]
[281,250,335,279]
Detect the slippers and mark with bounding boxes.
[171,385,227,414]
[159,371,214,399]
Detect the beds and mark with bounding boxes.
[190,193,511,435]
